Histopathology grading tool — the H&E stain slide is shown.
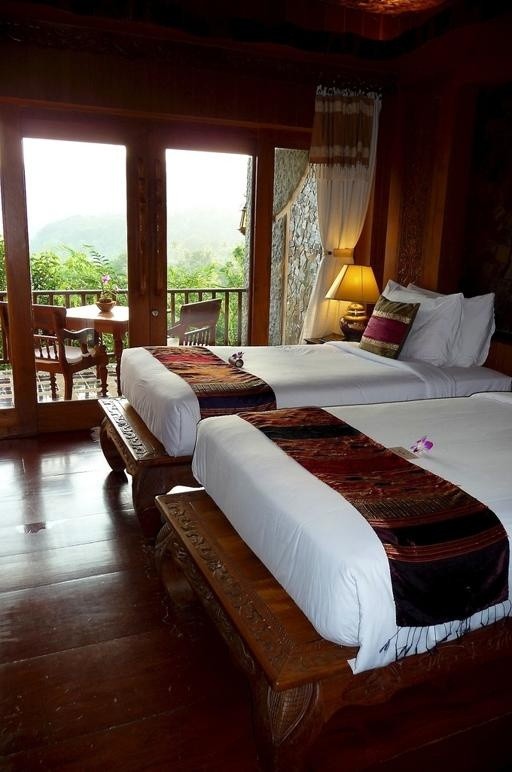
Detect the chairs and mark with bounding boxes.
[0,302,108,400]
[165,298,225,346]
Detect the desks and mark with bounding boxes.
[61,303,130,402]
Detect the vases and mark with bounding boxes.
[94,302,117,311]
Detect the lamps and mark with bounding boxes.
[322,265,383,340]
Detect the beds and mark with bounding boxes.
[95,323,510,510]
[143,386,511,760]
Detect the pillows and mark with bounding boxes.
[361,276,496,368]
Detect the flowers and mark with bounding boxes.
[95,272,115,301]
